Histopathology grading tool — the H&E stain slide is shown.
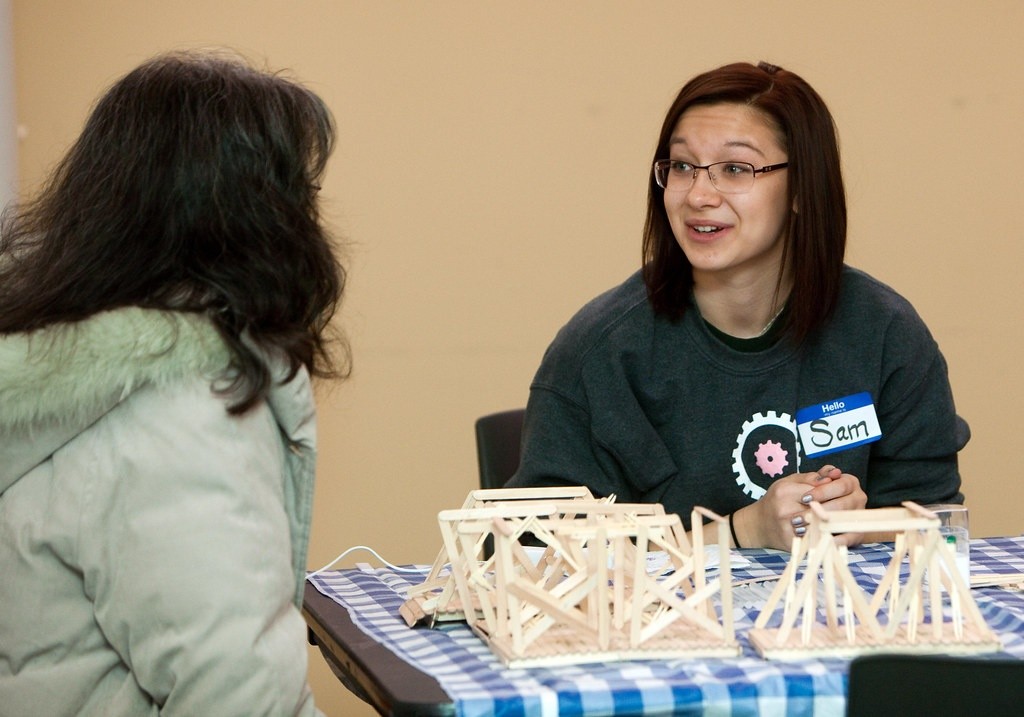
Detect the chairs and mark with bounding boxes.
[846,652,1024,717]
[473,409,526,561]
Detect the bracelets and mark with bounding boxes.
[729,511,742,549]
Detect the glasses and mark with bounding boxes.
[305,182,322,219]
[654,159,791,192]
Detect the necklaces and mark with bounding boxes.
[759,304,787,335]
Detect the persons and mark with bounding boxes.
[0,53,354,717]
[506,61,969,552]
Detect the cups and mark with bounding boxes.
[920,503,971,593]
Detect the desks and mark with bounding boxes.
[304,536,1024,717]
